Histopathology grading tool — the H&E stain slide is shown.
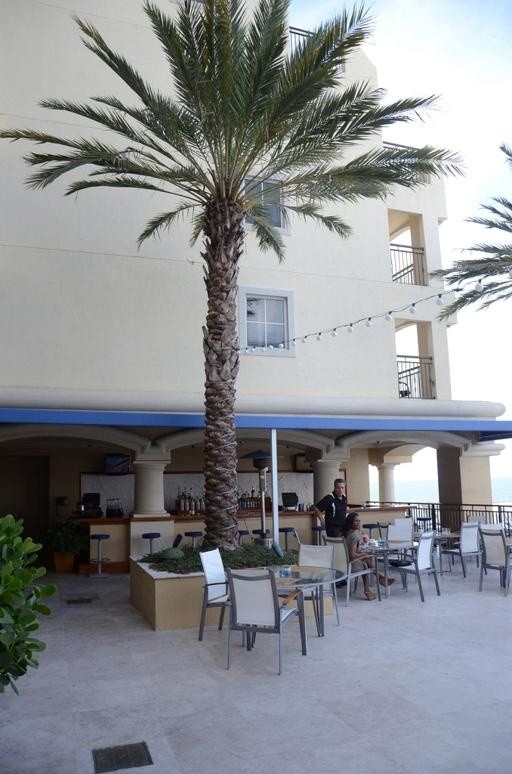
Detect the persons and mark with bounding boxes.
[340,511,395,600]
[312,479,349,588]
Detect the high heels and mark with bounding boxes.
[381,579,395,587]
[366,591,376,600]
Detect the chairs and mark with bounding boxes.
[297,543,340,636]
[322,516,512,606]
[198,549,232,641]
[222,568,307,674]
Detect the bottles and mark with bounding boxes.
[175,486,261,517]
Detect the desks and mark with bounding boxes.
[242,565,346,650]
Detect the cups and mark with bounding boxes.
[442,528,447,535]
[272,565,280,578]
[299,504,304,512]
[447,528,451,536]
[363,535,386,547]
[282,566,292,578]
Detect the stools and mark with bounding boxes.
[239,530,251,544]
[142,533,161,554]
[312,526,324,545]
[184,531,202,547]
[279,528,293,550]
[87,534,110,578]
[254,529,270,539]
